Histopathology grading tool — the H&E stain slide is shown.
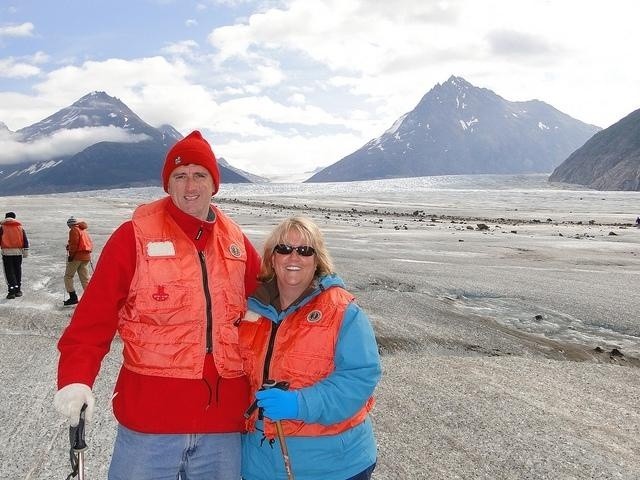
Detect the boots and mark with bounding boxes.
[7,283,23,299]
[63,291,79,306]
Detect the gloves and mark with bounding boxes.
[52,382,94,427]
[254,386,299,423]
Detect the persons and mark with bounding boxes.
[61,215,93,305]
[0,211,29,299]
[55,129,265,480]
[237,215,382,480]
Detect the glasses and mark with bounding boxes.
[272,244,317,257]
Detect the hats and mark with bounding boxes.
[67,216,77,225]
[162,130,220,196]
